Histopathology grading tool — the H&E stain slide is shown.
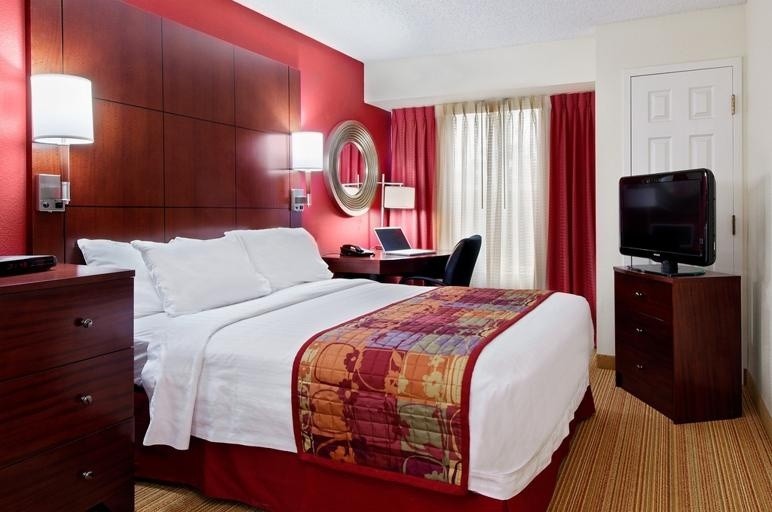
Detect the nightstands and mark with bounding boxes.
[0,265,135,512]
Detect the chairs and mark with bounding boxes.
[399,235,481,287]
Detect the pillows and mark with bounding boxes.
[133,234,269,317]
[225,228,332,292]
[77,239,162,320]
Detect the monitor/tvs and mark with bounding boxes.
[619,168,717,277]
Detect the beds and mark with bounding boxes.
[134,278,596,512]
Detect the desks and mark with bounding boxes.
[321,252,450,284]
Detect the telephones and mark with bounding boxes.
[340,244,373,257]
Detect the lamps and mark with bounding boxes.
[291,131,324,213]
[29,75,94,212]
[377,174,415,227]
[342,174,363,194]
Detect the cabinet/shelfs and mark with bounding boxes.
[614,266,742,423]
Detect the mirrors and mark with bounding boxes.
[323,119,377,217]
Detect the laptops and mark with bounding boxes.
[374,227,436,256]
